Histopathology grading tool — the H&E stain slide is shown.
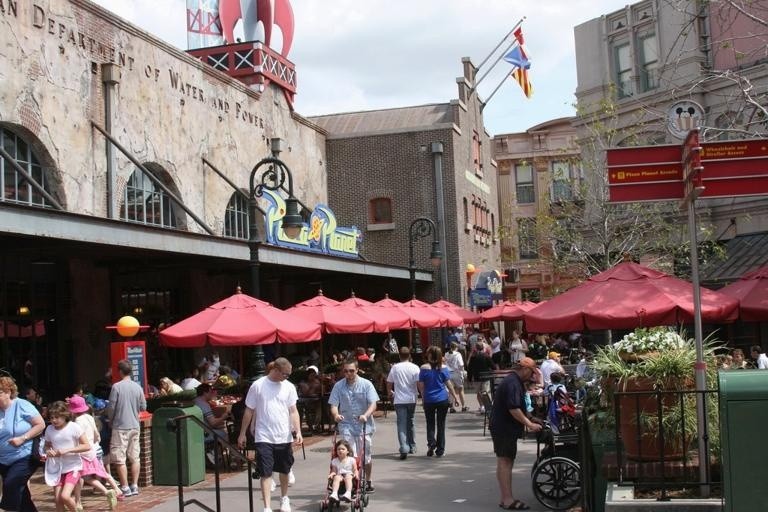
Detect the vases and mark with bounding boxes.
[618,350,686,365]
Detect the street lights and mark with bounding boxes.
[407,215,441,363]
[244,158,303,402]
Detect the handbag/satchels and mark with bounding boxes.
[550,400,561,426]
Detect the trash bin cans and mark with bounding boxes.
[152,405,205,486]
[715,368,768,512]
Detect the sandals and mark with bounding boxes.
[450,409,455,413]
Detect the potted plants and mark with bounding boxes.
[589,334,733,460]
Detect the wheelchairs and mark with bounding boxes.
[531,422,581,512]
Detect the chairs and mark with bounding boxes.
[226,421,248,469]
[491,384,499,400]
[477,392,494,436]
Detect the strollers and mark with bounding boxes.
[320,415,369,512]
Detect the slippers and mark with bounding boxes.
[498,498,528,510]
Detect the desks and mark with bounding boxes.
[159,394,253,468]
[298,392,330,435]
[525,388,547,436]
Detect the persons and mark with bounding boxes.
[716,346,767,373]
[238,357,303,512]
[149,325,600,470]
[326,359,378,504]
[0,359,148,511]
[489,358,541,511]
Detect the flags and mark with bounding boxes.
[513,25,524,45]
[503,45,531,69]
[512,67,535,96]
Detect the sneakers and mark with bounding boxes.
[330,494,339,500]
[435,448,445,458]
[343,495,351,500]
[130,486,139,495]
[264,508,271,512]
[107,490,117,508]
[400,453,407,459]
[280,496,291,512]
[366,483,376,493]
[462,406,470,412]
[426,438,436,457]
[207,452,216,465]
[121,487,131,496]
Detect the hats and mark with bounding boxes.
[549,351,560,358]
[517,357,538,374]
[306,365,319,375]
[44,458,62,486]
[67,396,89,414]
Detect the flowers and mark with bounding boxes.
[613,325,686,351]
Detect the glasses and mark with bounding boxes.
[311,374,314,376]
[343,368,355,373]
[478,338,484,340]
